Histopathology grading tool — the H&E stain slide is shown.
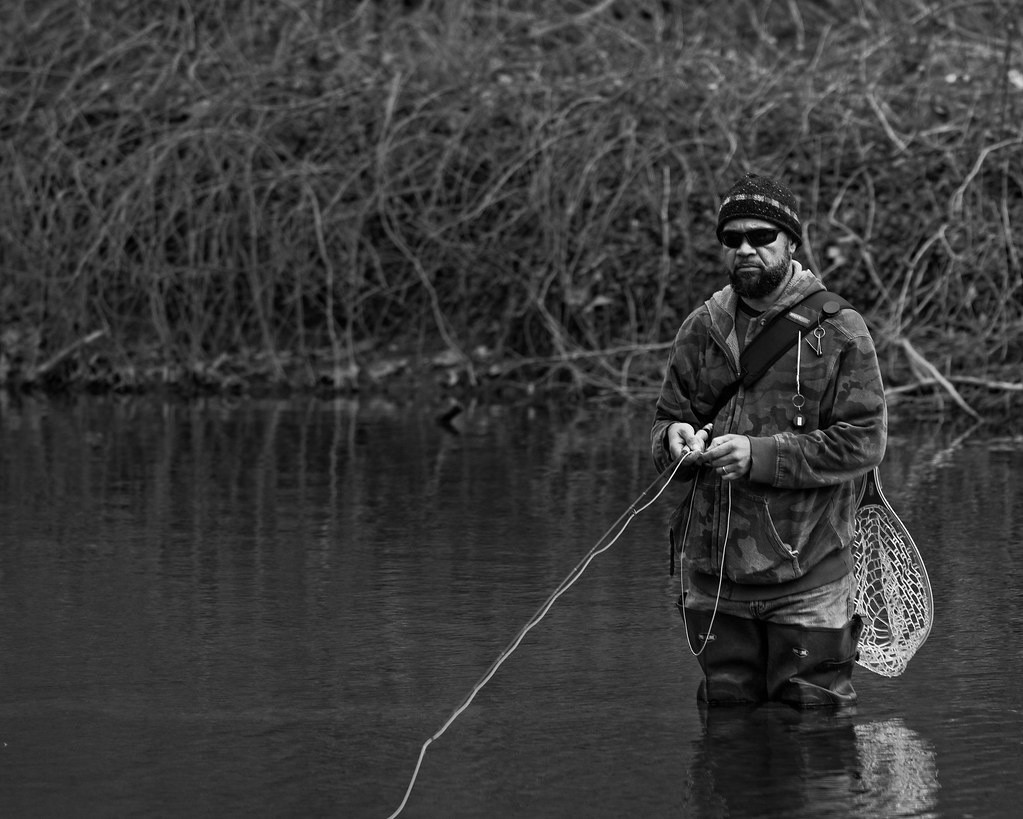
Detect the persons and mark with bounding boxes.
[650,173,888,710]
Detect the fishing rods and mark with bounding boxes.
[429,422,716,739]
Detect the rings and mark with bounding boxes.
[723,467,728,475]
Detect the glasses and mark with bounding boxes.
[721,226,784,248]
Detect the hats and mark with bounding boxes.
[716,174,802,248]
